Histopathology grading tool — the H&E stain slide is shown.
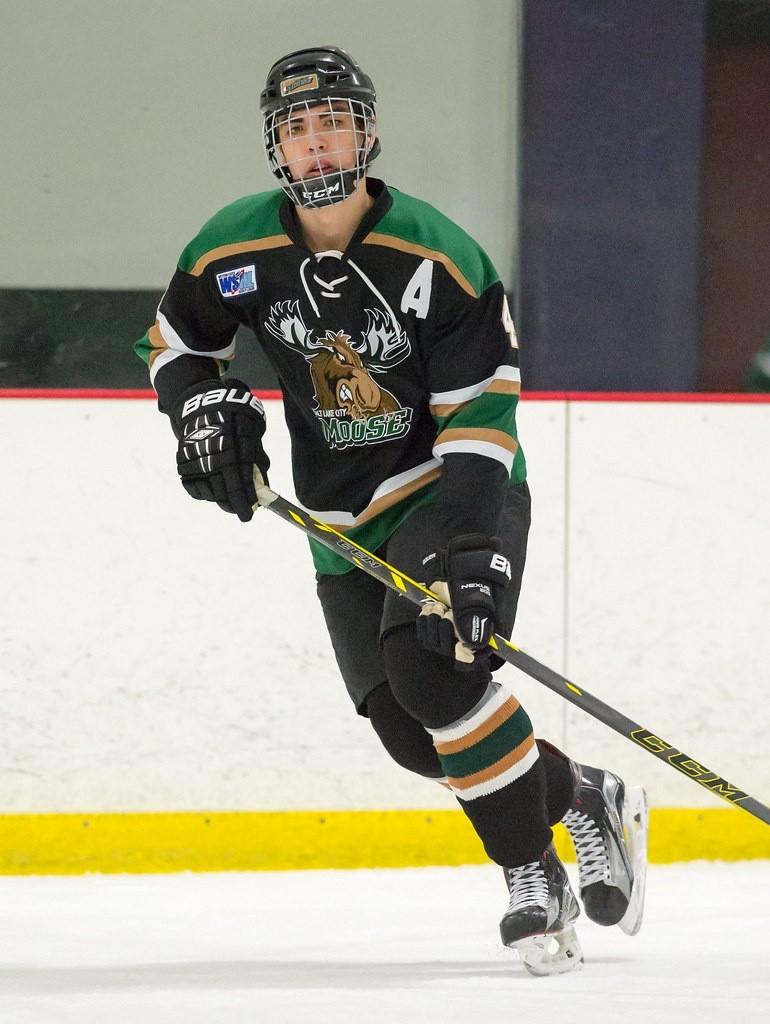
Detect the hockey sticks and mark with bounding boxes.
[252,467,770,828]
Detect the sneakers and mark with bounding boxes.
[499,838,581,974]
[561,756,648,938]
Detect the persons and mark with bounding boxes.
[134,45,649,975]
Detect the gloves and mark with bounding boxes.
[169,378,270,522]
[417,532,511,668]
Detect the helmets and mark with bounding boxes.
[259,45,381,211]
